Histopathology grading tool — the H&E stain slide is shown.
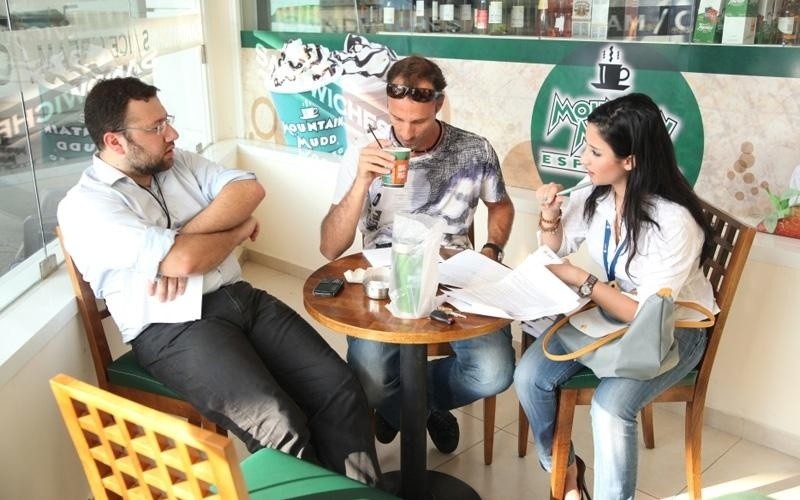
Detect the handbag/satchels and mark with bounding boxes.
[541,284,716,384]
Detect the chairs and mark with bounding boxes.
[514,192,758,500]
[361,209,496,468]
[54,221,231,446]
[48,373,402,500]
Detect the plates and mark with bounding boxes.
[592,83,630,91]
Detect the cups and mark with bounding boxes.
[269,61,348,152]
[390,236,429,319]
[380,148,412,188]
[598,62,630,85]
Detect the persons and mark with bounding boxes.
[319,55,516,455]
[56,77,386,492]
[512,93,721,500]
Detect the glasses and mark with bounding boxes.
[385,85,445,102]
[110,115,176,135]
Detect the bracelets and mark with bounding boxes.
[538,218,561,234]
[540,209,562,223]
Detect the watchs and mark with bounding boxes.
[578,275,598,297]
[483,243,504,262]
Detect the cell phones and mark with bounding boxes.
[312,279,344,296]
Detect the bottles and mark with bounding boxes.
[775,1,799,46]
[381,0,571,38]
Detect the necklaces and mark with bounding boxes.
[136,172,172,232]
[389,119,442,158]
[602,221,631,282]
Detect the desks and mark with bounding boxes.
[301,244,514,500]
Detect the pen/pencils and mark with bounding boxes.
[437,307,467,318]
[555,181,592,197]
